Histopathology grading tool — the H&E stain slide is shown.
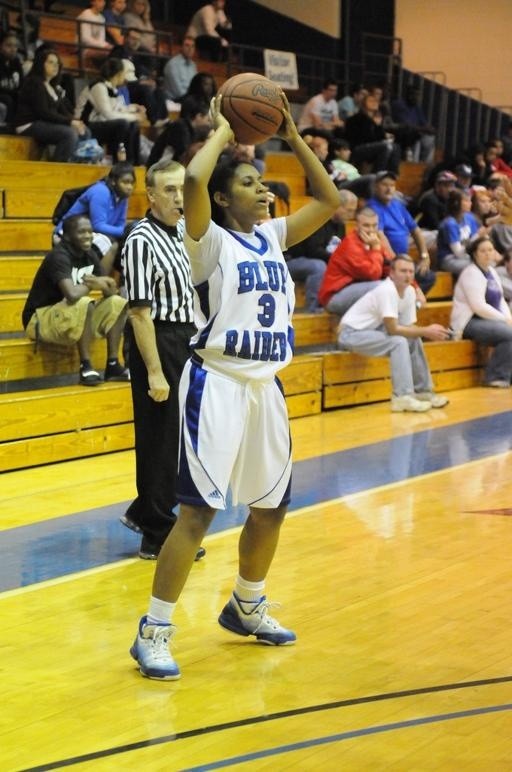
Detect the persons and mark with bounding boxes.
[285,82,511,410]
[1,1,269,174]
[20,137,276,382]
[126,89,345,681]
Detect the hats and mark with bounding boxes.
[456,164,474,176]
[438,171,458,181]
[122,59,137,82]
[375,170,398,181]
[470,184,490,195]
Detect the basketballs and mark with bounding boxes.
[215,72,285,145]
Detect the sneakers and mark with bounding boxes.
[130,615,181,680]
[80,370,102,386]
[104,367,131,382]
[218,590,297,646]
[416,392,449,408]
[304,302,325,313]
[483,380,510,388]
[391,395,432,412]
[151,118,172,128]
[140,546,206,561]
[120,514,143,534]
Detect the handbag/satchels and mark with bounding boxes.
[73,130,104,158]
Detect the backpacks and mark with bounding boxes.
[52,183,99,226]
